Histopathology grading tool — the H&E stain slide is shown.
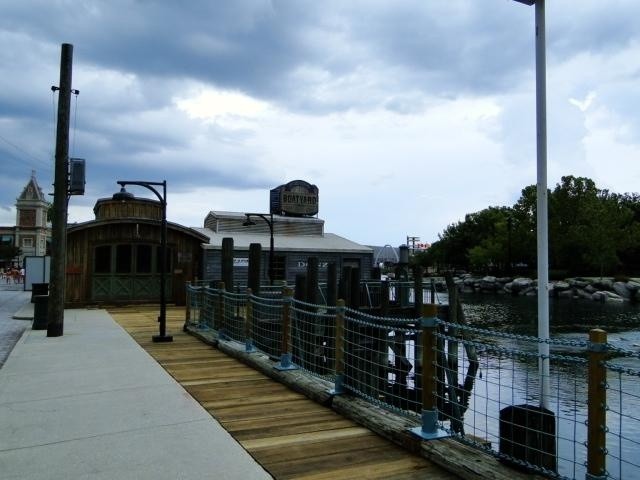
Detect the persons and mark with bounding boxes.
[0,266,25,286]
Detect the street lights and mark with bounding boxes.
[113,178,175,344]
[397,243,413,282]
[242,210,277,286]
